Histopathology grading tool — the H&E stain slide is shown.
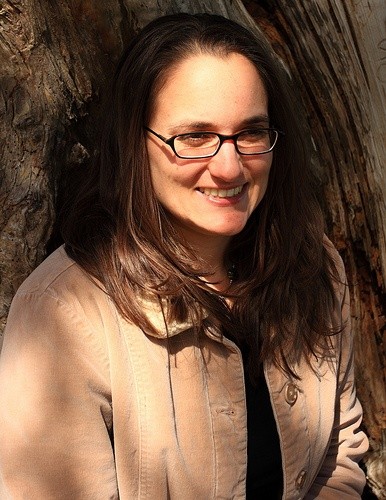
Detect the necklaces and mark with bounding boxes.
[189,264,237,300]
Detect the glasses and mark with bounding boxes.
[143,125,278,159]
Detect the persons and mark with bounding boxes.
[0,12,370,500]
